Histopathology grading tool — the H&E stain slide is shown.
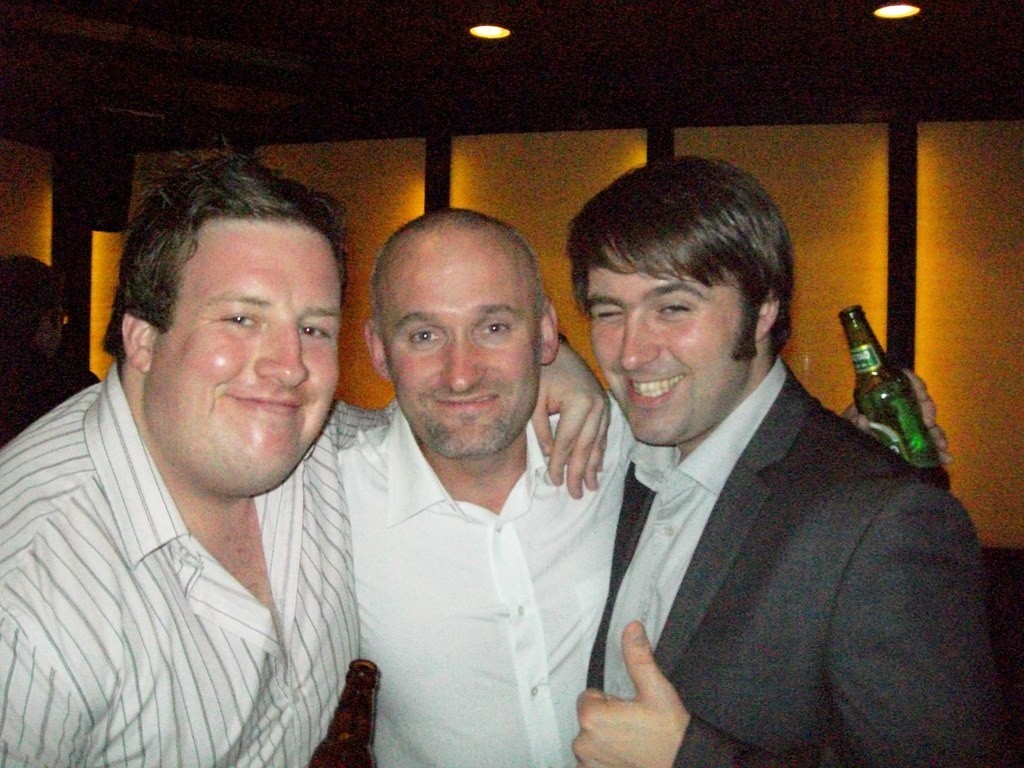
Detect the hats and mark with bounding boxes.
[0,254,77,359]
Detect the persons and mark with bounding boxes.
[0,149,613,768]
[331,208,955,768]
[0,256,86,447]
[567,153,1010,768]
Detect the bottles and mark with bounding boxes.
[837,303,952,493]
[307,658,381,768]
